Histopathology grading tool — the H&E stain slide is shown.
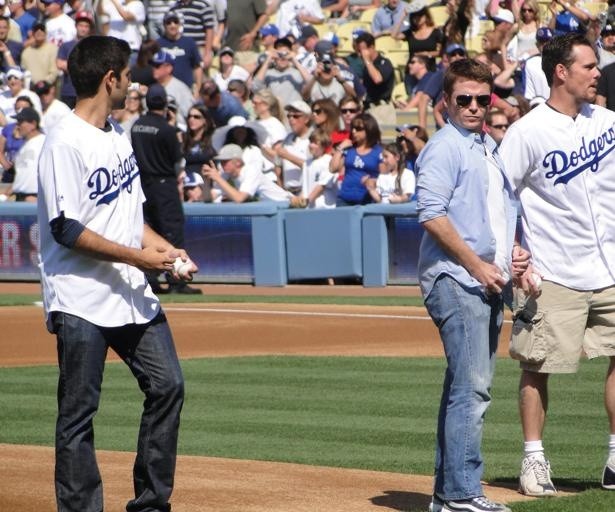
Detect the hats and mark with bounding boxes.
[600,23,614,35]
[445,43,465,56]
[11,107,40,122]
[75,10,95,25]
[146,10,376,161]
[6,68,24,81]
[536,27,553,43]
[489,8,514,24]
[553,10,585,36]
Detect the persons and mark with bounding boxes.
[498,30,614,497]
[130,83,203,295]
[36,35,198,511]
[0,1,614,205]
[414,60,531,512]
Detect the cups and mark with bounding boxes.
[381,192,389,204]
[211,189,223,204]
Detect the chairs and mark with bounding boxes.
[208,0,609,144]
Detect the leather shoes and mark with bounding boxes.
[147,284,168,293]
[166,284,202,295]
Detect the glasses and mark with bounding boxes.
[455,94,491,107]
[492,123,508,128]
[521,8,531,13]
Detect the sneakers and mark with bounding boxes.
[428,495,442,512]
[440,495,512,512]
[517,452,558,497]
[600,456,615,489]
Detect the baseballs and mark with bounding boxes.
[169,257,190,274]
[528,274,542,290]
